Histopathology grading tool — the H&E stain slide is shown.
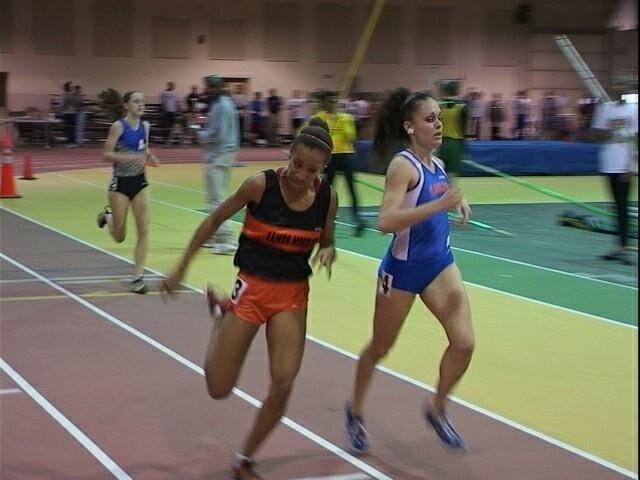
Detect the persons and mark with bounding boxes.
[296,89,367,239]
[157,80,184,147]
[244,88,383,149]
[61,79,84,149]
[343,85,476,458]
[73,85,87,148]
[186,73,240,255]
[467,86,597,141]
[578,80,639,266]
[160,117,341,480]
[187,83,201,108]
[436,80,471,184]
[96,86,160,294]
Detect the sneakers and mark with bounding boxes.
[97,205,113,227]
[423,404,465,450]
[129,275,150,293]
[207,286,228,318]
[230,453,262,479]
[344,400,369,455]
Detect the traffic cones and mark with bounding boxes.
[18,152,38,180]
[0,128,22,199]
